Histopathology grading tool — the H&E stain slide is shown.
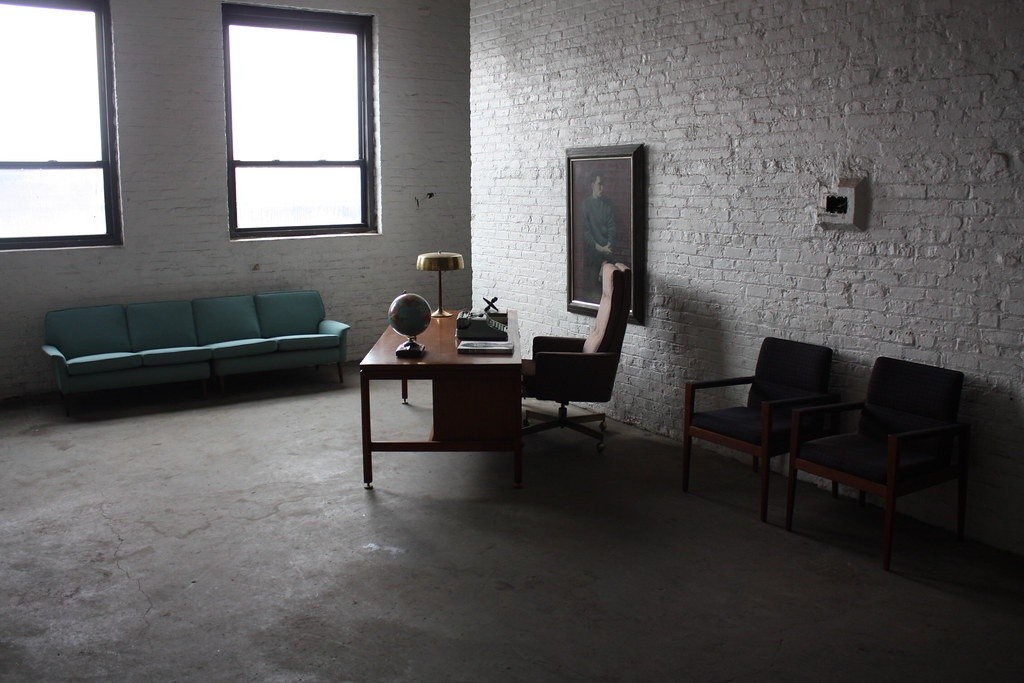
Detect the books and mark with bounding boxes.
[457,340,514,354]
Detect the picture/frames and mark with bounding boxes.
[567,143,646,326]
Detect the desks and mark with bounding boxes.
[359,310,523,490]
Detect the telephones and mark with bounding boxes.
[456,310,509,341]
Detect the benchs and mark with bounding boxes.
[42,291,351,417]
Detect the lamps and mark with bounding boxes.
[416,251,464,317]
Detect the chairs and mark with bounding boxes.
[683,337,845,523]
[786,356,970,572]
[520,263,631,451]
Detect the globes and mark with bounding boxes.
[387,289,431,359]
